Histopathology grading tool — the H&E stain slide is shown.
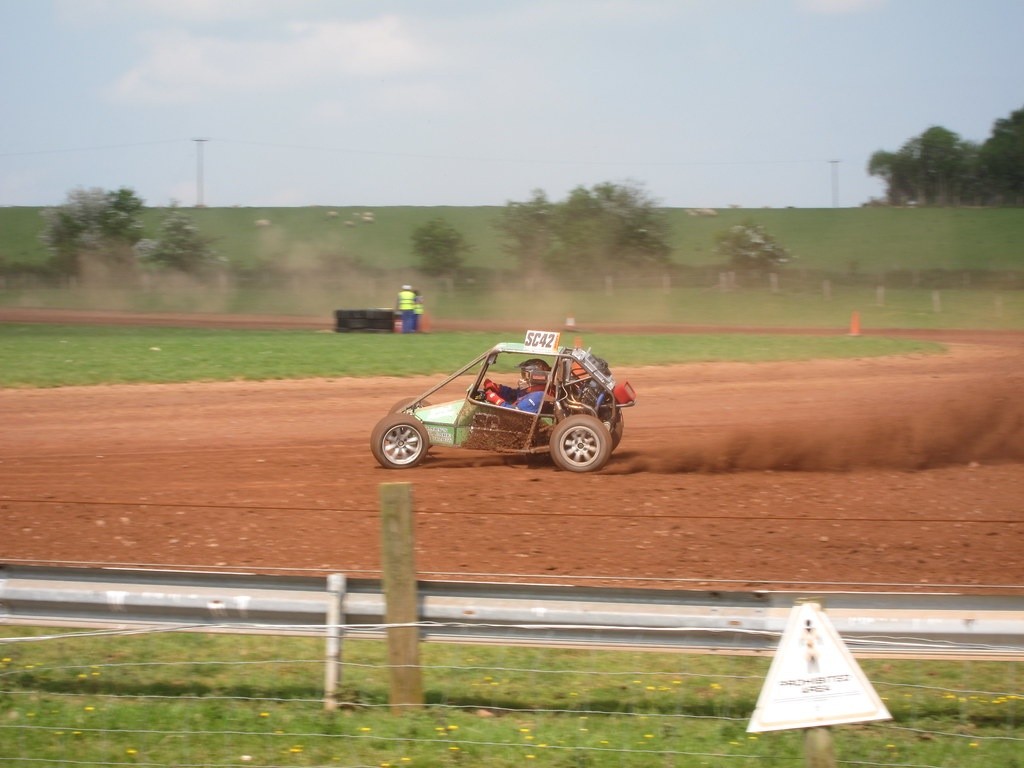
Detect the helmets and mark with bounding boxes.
[516,358,553,393]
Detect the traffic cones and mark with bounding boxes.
[844,307,863,337]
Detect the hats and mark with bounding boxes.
[401,284,412,291]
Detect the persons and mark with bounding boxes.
[396,284,424,333]
[484,359,554,413]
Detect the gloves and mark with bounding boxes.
[483,390,506,406]
[485,379,502,394]
